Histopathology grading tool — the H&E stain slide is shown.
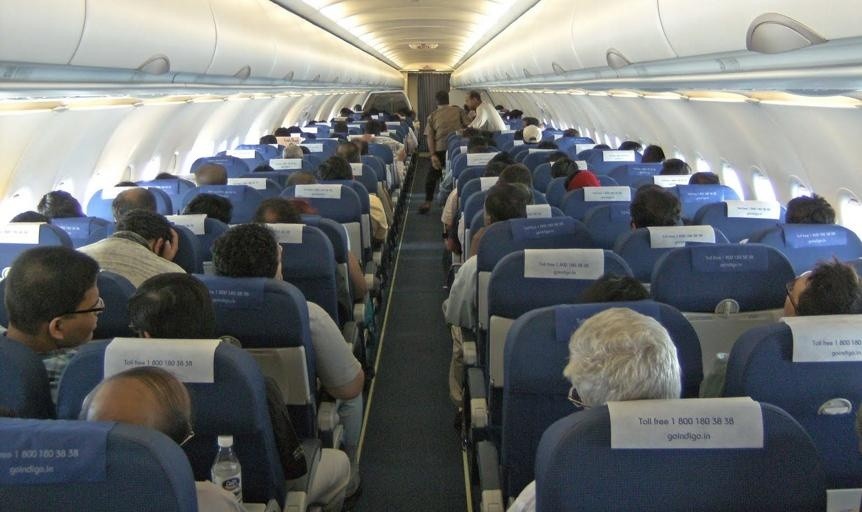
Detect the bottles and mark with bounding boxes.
[211,435,242,507]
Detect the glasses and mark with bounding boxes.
[179,422,194,447]
[47,298,106,324]
[785,277,803,316]
[567,386,591,409]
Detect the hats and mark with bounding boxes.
[564,170,601,192]
[522,125,543,144]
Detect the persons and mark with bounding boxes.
[579,272,651,301]
[215,224,364,501]
[782,192,837,225]
[504,308,685,512]
[131,274,352,511]
[74,208,188,285]
[696,253,862,401]
[0,247,106,419]
[292,199,370,304]
[9,89,721,227]
[77,367,244,512]
[443,182,538,431]
[253,196,348,330]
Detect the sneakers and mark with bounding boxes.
[419,202,430,215]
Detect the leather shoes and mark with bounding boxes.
[341,487,362,512]
